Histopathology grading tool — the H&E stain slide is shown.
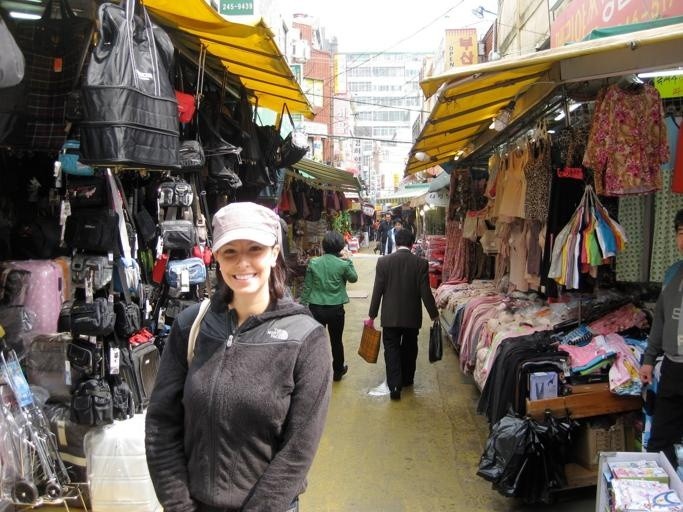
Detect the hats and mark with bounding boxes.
[212,202,281,253]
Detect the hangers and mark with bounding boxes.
[597,73,661,96]
[508,112,593,155]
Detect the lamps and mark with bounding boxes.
[472,5,497,19]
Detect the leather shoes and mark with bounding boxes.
[334,366,347,381]
[391,387,400,399]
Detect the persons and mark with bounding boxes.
[368,229,441,400]
[299,233,358,381]
[145,202,335,512]
[370,214,417,256]
[638,208,683,472]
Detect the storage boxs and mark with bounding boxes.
[575,416,627,472]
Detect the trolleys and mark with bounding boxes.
[1,344,76,508]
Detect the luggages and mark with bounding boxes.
[27,333,75,403]
[44,406,91,508]
[0,260,64,357]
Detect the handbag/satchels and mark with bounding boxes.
[430,326,443,362]
[0,0,182,176]
[175,51,307,193]
[358,325,381,363]
[60,177,163,425]
[149,172,212,325]
